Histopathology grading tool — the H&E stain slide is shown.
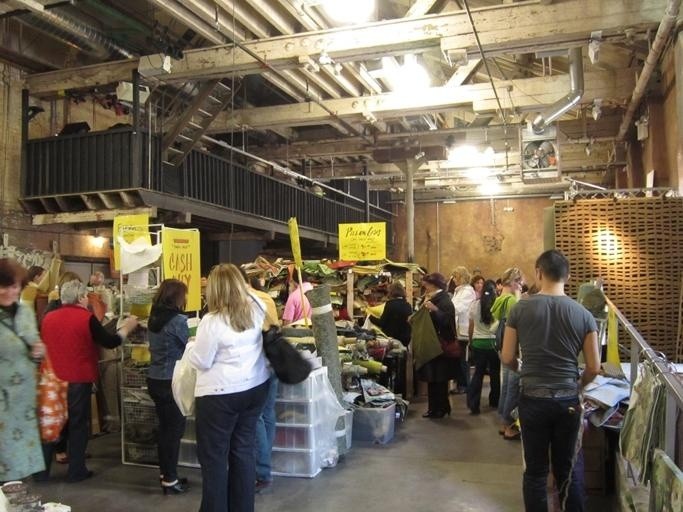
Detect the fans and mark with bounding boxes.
[520,126,561,184]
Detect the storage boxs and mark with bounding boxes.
[344,400,397,446]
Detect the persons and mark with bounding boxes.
[501,250,601,512]
[1,255,47,489]
[187,262,272,511]
[20,264,139,483]
[381,264,539,442]
[238,266,279,493]
[281,267,314,326]
[143,276,190,497]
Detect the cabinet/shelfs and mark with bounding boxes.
[195,269,431,403]
[118,265,333,480]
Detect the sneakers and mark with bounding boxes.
[499,425,521,440]
[55,449,94,483]
[450,387,464,394]
[256,479,273,492]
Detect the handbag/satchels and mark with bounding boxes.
[172,358,197,417]
[261,324,312,385]
[495,320,505,351]
[37,372,70,443]
[409,305,444,370]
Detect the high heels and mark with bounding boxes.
[159,475,192,495]
[422,405,451,419]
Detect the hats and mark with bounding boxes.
[423,272,447,290]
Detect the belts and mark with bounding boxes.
[526,388,578,399]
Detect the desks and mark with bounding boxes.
[589,409,626,430]
